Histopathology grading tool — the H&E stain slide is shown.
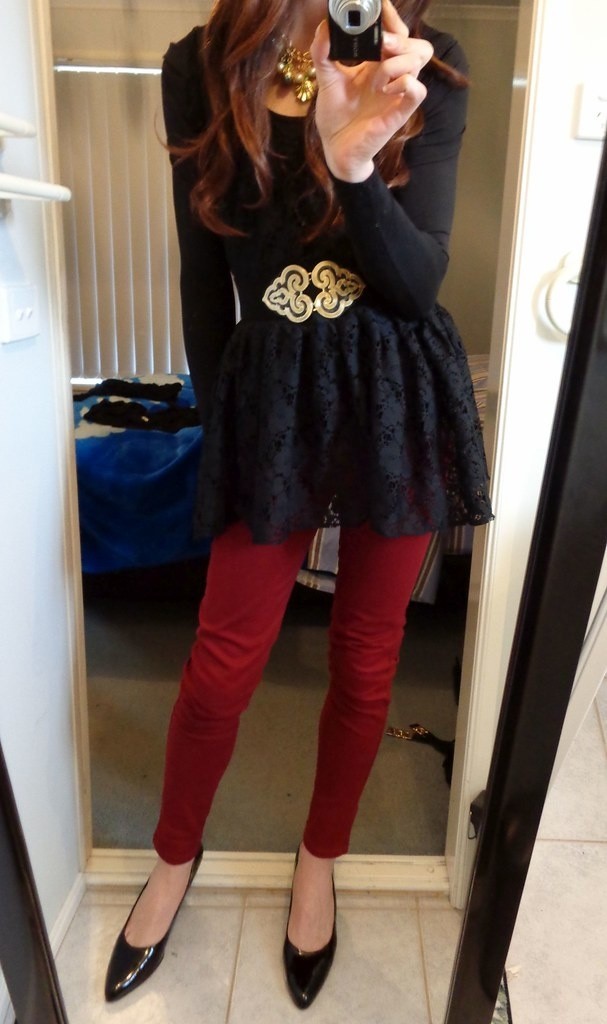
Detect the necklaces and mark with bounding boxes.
[269,26,320,103]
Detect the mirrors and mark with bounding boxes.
[0,0,607,1024]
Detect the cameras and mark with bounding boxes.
[328,0,383,62]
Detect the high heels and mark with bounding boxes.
[105,842,204,1001]
[282,841,337,1011]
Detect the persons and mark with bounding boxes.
[104,0,495,1011]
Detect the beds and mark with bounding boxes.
[73,352,491,626]
